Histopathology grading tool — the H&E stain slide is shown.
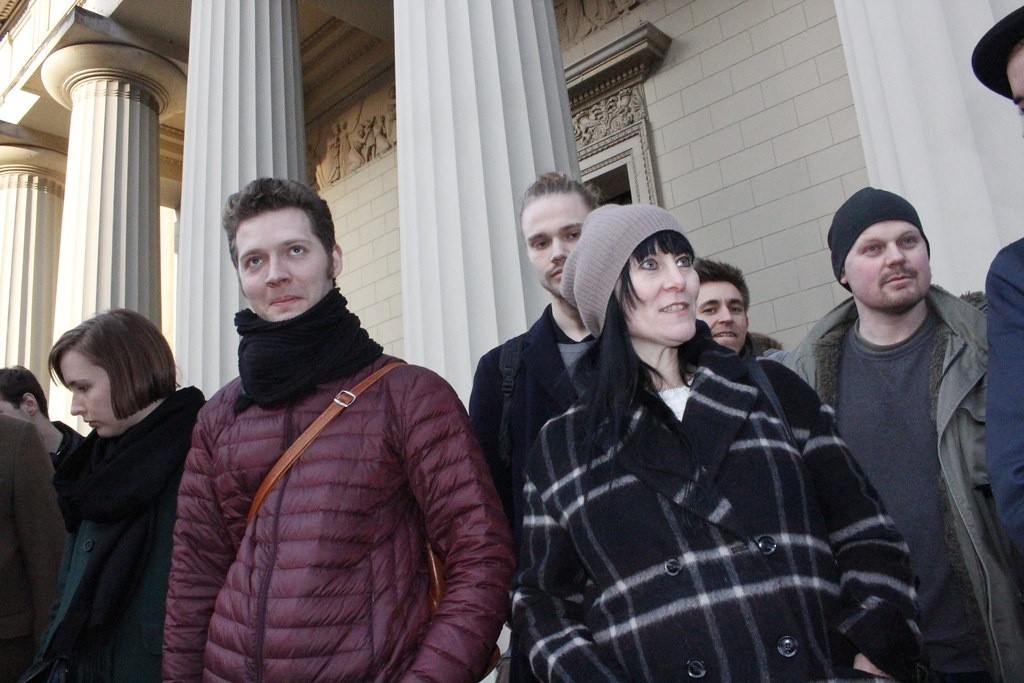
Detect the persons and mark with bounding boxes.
[968,3,1023,570]
[509,205,920,683]
[471,172,713,562]
[162,175,516,683]
[20,311,207,683]
[693,256,781,361]
[0,363,86,683]
[756,185,1024,682]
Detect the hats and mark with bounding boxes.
[560,204,687,340]
[828,187,930,293]
[972,6,1024,99]
